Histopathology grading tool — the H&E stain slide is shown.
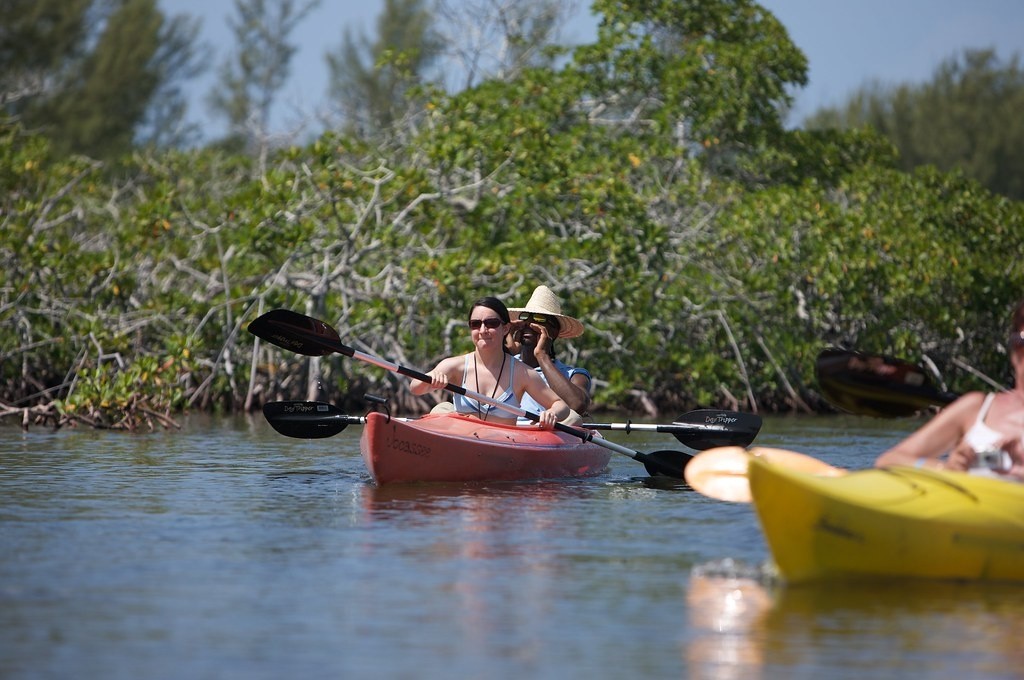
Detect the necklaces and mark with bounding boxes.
[474,351,506,421]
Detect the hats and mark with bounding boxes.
[507,285,584,338]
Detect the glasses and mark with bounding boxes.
[519,312,556,328]
[469,317,506,329]
[1008,330,1024,348]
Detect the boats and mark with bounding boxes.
[746,445,1023,583]
[358,410,614,486]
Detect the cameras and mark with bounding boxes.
[974,450,1002,468]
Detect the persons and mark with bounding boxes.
[408,285,592,431]
[874,304,1024,483]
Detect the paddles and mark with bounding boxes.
[263,401,762,452]
[682,445,750,507]
[246,308,692,484]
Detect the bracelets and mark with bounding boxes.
[914,457,927,468]
